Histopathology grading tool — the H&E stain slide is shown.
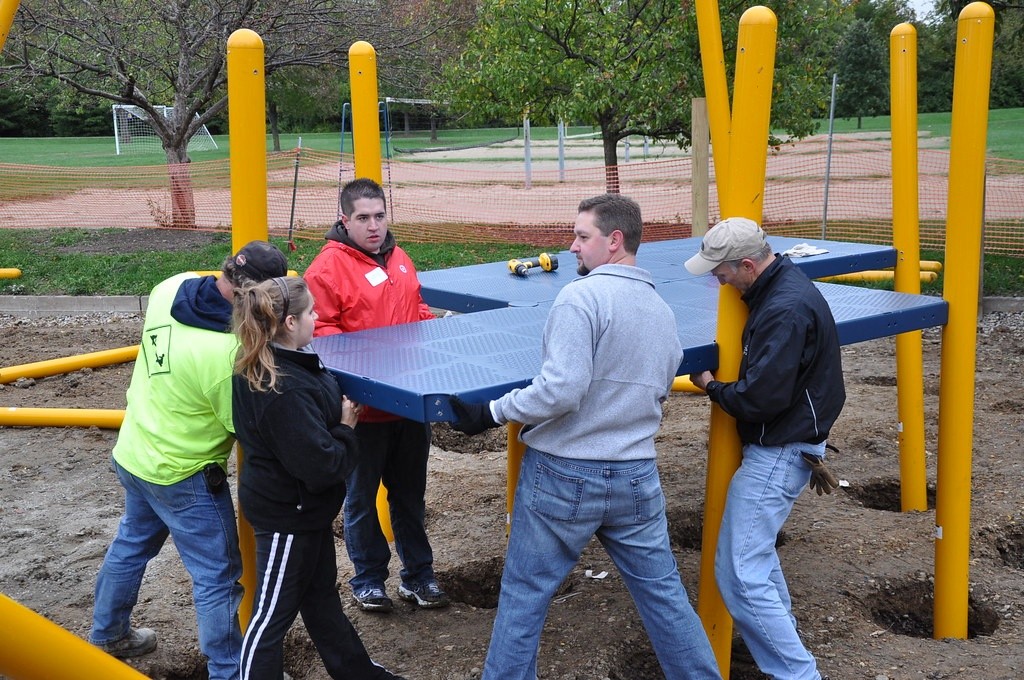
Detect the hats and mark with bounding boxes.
[685,217,766,275]
[231,241,288,284]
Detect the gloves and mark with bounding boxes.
[448,395,501,436]
[801,451,839,496]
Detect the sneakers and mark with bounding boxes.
[89,627,158,658]
[352,588,392,611]
[397,580,450,608]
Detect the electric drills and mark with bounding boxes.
[508,253,559,277]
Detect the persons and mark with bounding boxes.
[684,218,846,680]
[231,278,407,680]
[91,240,290,680]
[302,179,452,607]
[448,192,723,680]
